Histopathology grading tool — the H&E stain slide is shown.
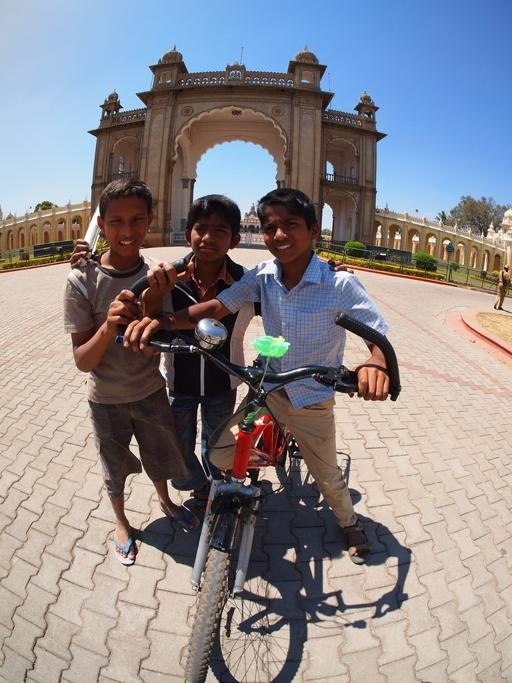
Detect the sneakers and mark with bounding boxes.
[498,307,503,310]
[494,305,496,309]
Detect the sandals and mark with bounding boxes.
[343,518,370,564]
[194,484,210,515]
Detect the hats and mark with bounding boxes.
[504,265,509,270]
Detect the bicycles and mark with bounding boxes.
[116,311,401,683]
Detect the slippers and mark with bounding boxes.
[111,526,134,565]
[174,505,201,531]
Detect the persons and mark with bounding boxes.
[122,186,398,565]
[493,263,511,309]
[62,179,199,566]
[70,193,354,507]
